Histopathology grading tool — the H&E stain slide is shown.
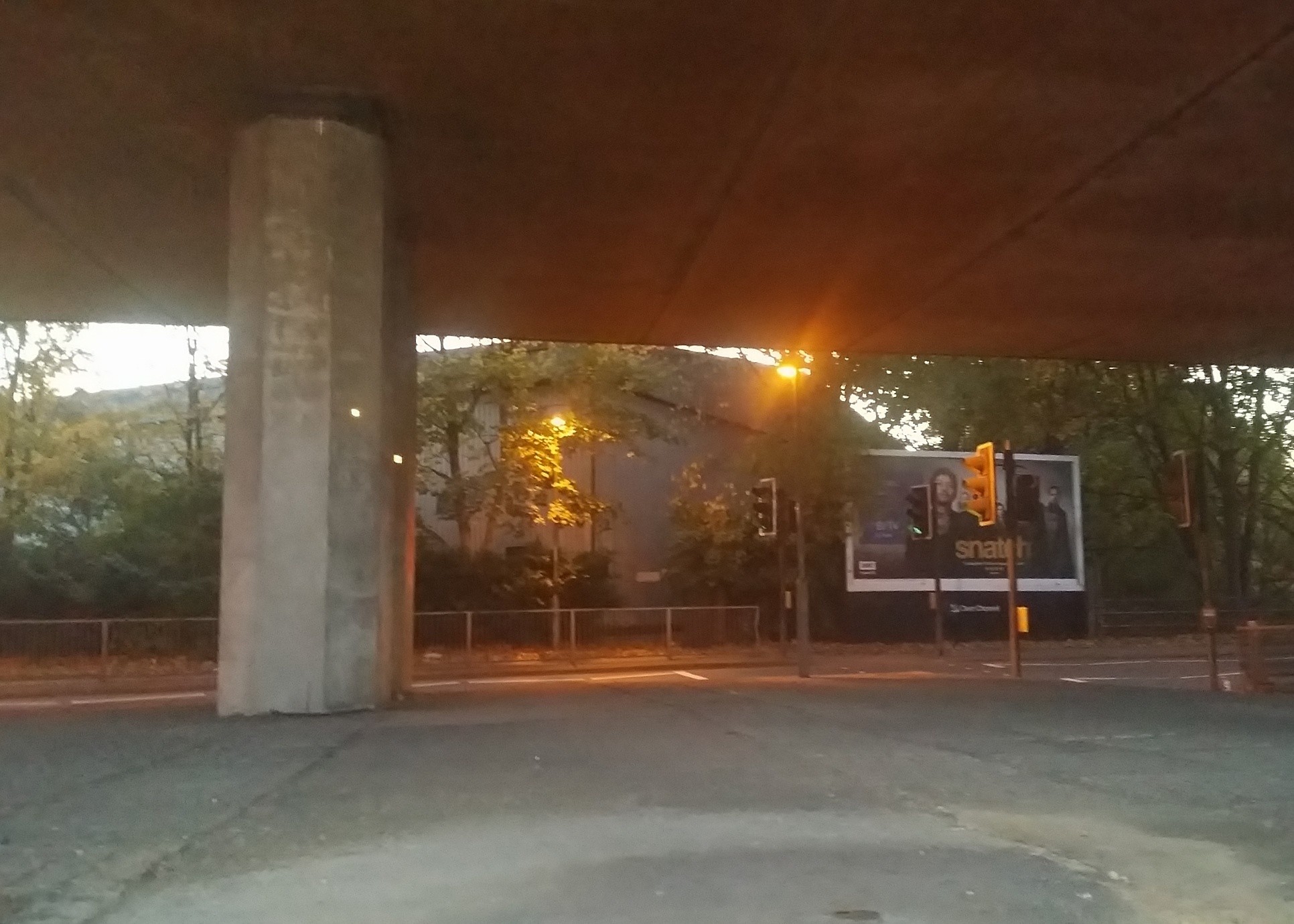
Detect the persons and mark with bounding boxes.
[898,468,1075,579]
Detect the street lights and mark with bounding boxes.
[549,413,565,649]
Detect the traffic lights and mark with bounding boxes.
[1161,448,1192,531]
[905,484,934,541]
[960,441,997,527]
[751,477,777,536]
